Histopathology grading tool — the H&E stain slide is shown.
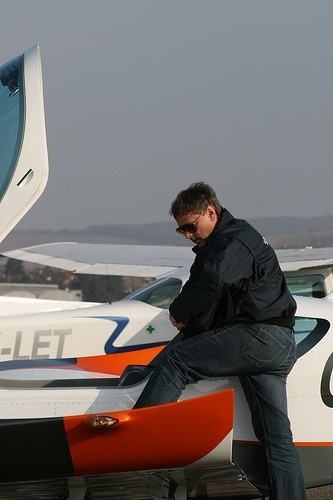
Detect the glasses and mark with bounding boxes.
[175,206,209,236]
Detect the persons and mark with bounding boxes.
[132,182,306,500]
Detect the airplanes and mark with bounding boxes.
[1,241,332,495]
